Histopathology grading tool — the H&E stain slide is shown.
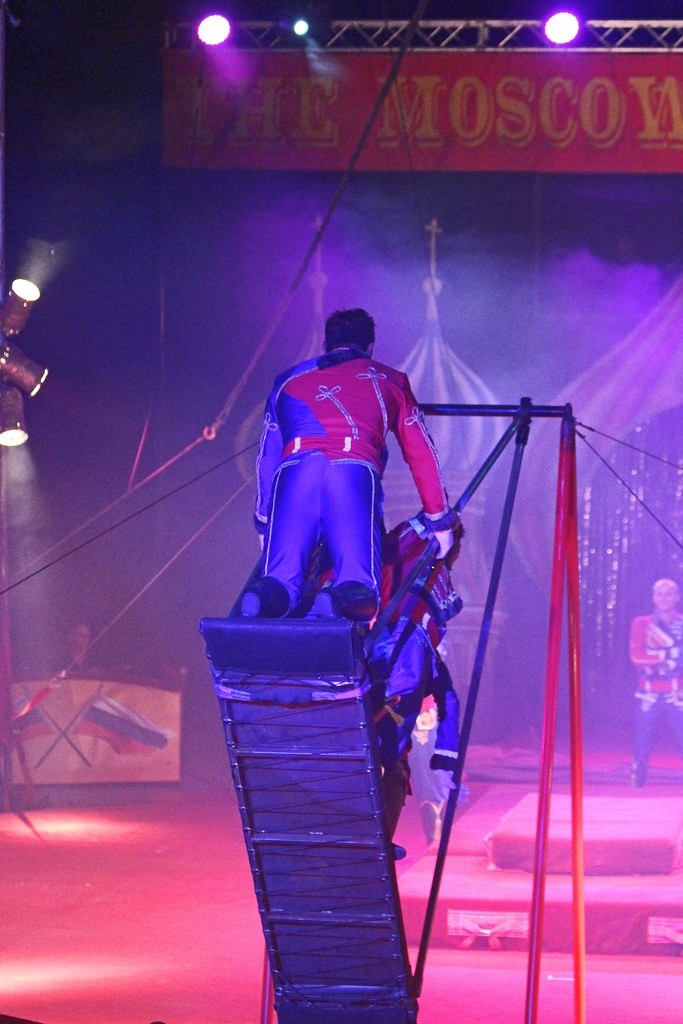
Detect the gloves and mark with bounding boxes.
[430,509,455,560]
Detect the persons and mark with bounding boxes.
[628,578,683,790]
[241,308,460,629]
[368,514,466,860]
[51,626,110,682]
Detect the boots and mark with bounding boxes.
[314,580,379,622]
[239,576,291,619]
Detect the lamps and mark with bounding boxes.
[0,385,29,447]
[0,341,48,398]
[0,278,42,338]
